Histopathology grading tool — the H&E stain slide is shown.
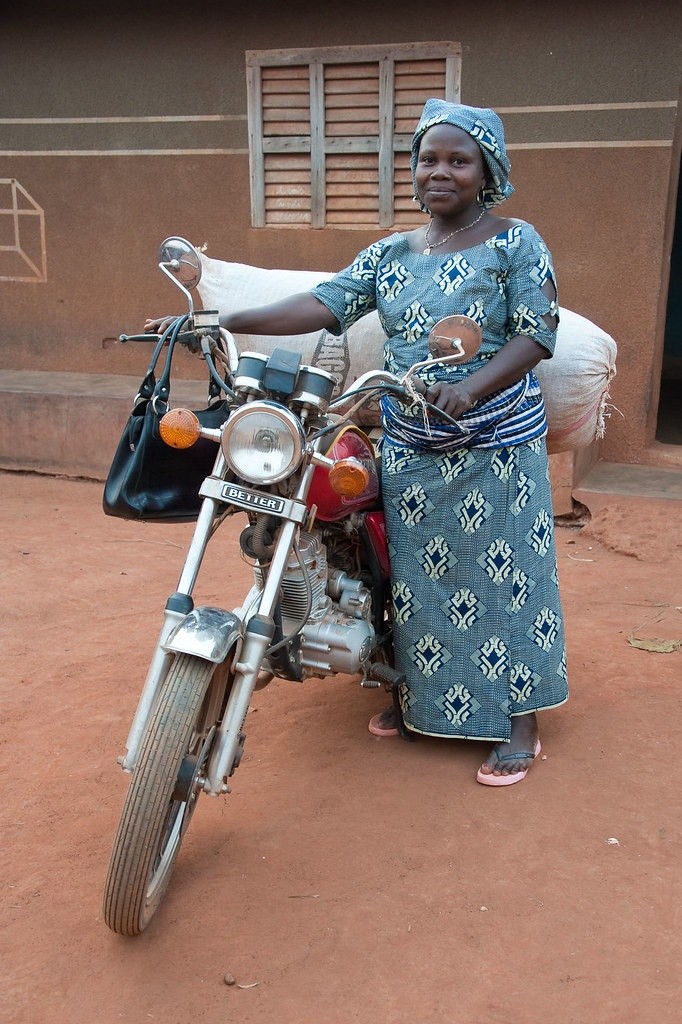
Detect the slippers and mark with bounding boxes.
[476,739,542,786]
[368,708,411,736]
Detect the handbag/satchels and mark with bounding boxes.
[104,314,230,524]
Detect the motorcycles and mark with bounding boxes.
[103,237,483,934]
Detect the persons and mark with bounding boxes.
[144,98,567,785]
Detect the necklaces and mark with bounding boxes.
[424,209,485,255]
